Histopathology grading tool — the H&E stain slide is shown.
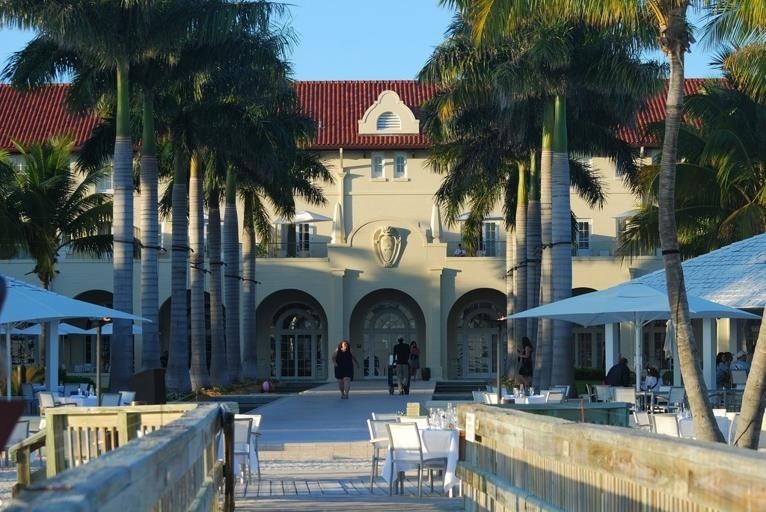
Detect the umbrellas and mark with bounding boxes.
[612,209,644,219]
[449,210,503,249]
[272,210,334,250]
[496,281,765,413]
[1,275,152,399]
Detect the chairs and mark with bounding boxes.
[472,373,765,446]
[0,381,262,495]
[373,413,396,420]
[368,419,404,497]
[386,422,453,498]
[399,416,429,429]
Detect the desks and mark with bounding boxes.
[383,427,461,493]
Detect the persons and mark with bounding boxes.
[393,337,410,395]
[716,352,753,392]
[408,341,421,382]
[646,369,663,413]
[605,359,632,387]
[454,242,466,256]
[332,339,356,399]
[640,367,651,411]
[518,336,536,389]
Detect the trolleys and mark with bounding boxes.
[387,359,412,396]
